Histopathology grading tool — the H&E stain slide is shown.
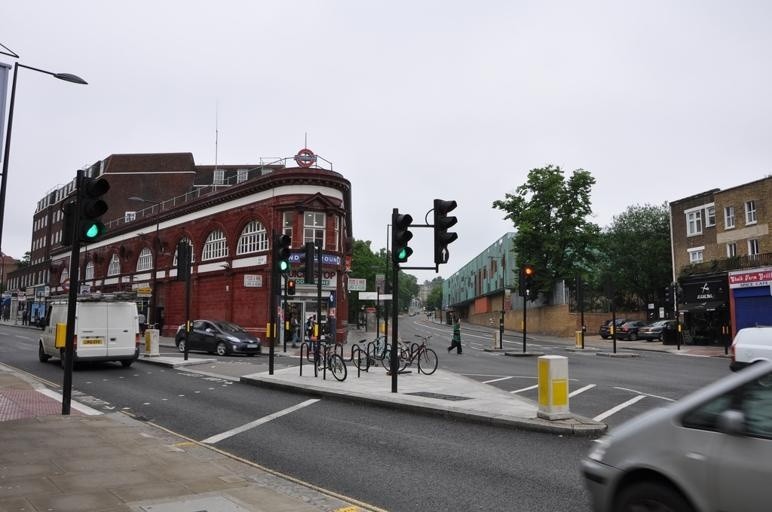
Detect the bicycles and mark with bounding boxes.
[398,334,439,376]
[311,335,348,382]
[352,337,402,373]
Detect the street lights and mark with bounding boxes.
[0,61,90,255]
[487,256,507,333]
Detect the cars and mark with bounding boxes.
[616,319,647,341]
[408,309,432,317]
[637,319,677,343]
[599,318,629,341]
[725,321,772,388]
[173,317,263,358]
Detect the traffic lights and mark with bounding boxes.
[287,280,296,296]
[81,176,111,248]
[432,198,458,264]
[299,241,315,284]
[518,263,535,297]
[276,233,292,274]
[394,213,414,264]
[665,286,672,306]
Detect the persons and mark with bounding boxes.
[446,317,463,355]
[22,308,28,325]
[306,316,318,351]
[139,311,146,337]
[290,314,300,348]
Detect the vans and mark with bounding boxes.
[37,290,144,368]
[576,352,772,511]
